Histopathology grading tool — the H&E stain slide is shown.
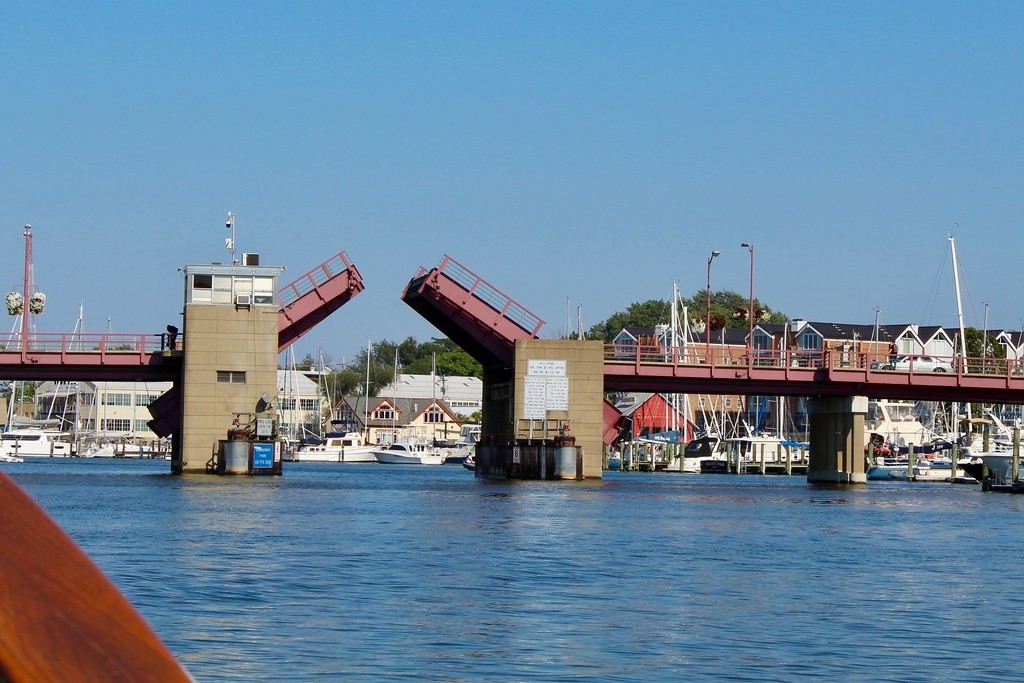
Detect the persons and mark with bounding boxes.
[887,339,898,370]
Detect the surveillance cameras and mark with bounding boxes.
[226,221,231,228]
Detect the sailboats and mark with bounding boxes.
[283,344,417,462]
[603,281,1024,494]
[0,299,172,461]
[428,350,471,462]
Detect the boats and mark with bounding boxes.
[463,447,475,470]
[373,443,448,465]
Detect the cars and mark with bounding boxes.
[870,352,956,374]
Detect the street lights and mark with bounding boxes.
[740,240,755,378]
[225,210,236,266]
[981,300,991,375]
[22,221,34,348]
[705,250,722,367]
